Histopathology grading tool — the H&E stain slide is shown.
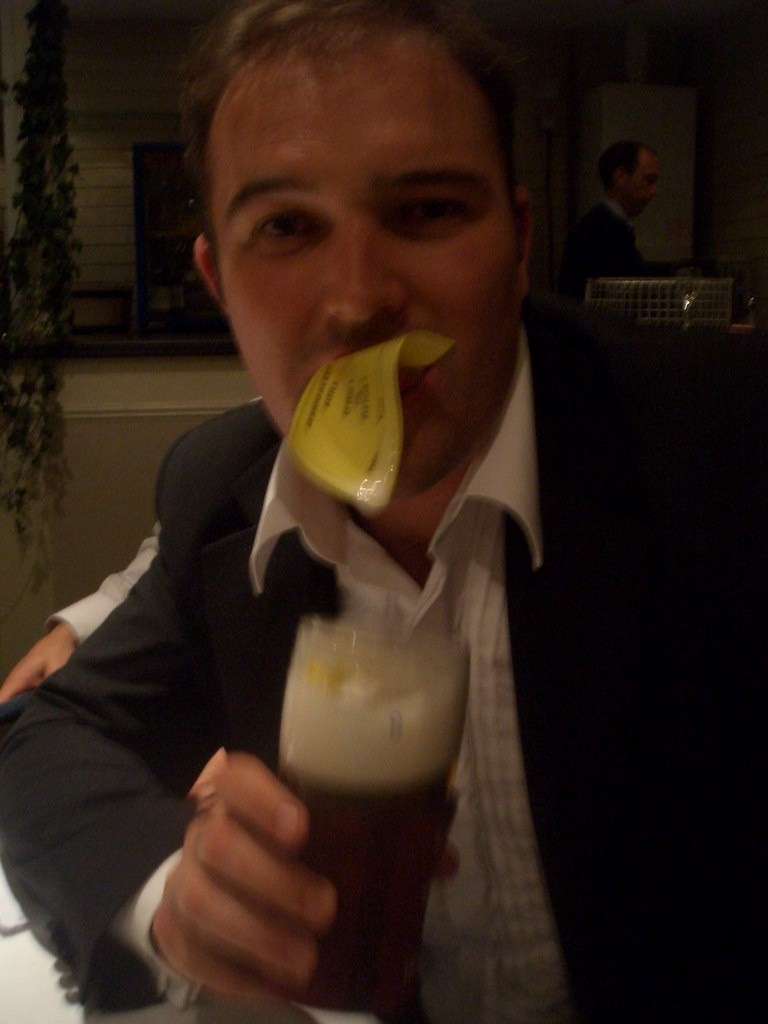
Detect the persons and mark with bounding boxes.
[0,0,768,1024]
[557,140,660,299]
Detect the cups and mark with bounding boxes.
[277,616,470,1014]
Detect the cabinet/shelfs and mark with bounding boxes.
[131,142,215,326]
